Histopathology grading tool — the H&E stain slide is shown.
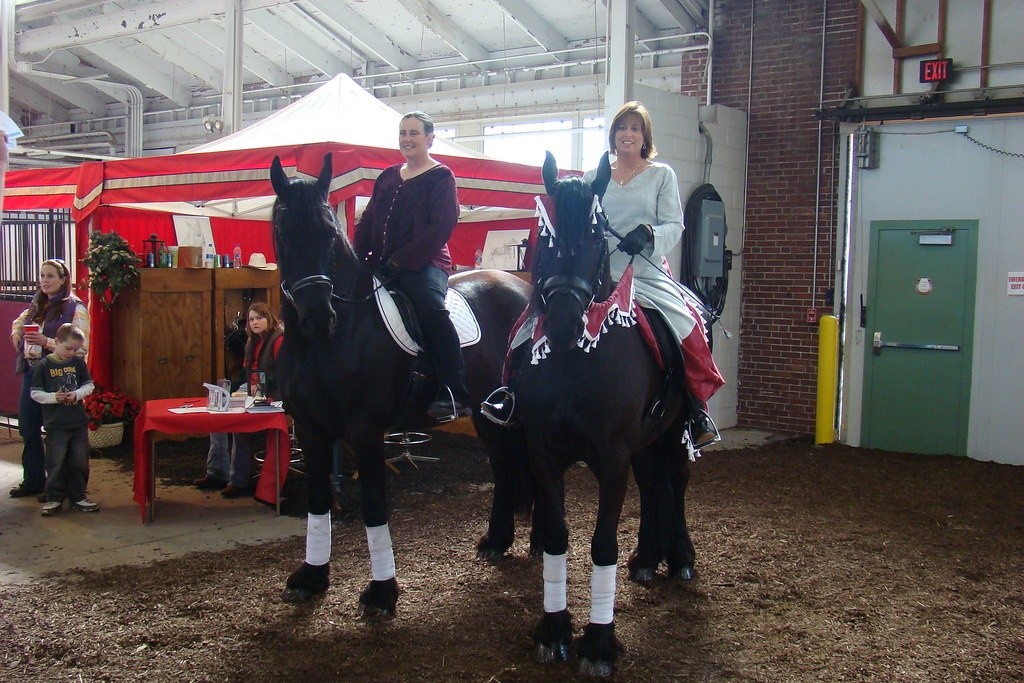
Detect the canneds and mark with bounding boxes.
[214,255,221,267]
[147,252,154,268]
[167,253,172,268]
[223,254,230,268]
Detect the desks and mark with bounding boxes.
[133,397,291,522]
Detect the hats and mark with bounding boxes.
[244,253,277,270]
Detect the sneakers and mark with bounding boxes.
[9,484,45,497]
[40,502,62,516]
[72,498,100,512]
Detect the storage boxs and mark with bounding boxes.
[176,246,203,269]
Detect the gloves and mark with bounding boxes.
[617,223,651,255]
[382,257,402,281]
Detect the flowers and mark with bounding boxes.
[81,381,142,431]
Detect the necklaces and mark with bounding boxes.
[405,166,422,181]
[616,159,643,189]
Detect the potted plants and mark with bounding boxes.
[78,232,142,313]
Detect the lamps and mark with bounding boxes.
[204,121,214,133]
[214,120,224,133]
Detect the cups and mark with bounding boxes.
[23,325,39,332]
[168,246,179,268]
[250,369,266,397]
[209,379,231,412]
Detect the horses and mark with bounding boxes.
[270,151,713,681]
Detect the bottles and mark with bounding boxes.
[233,243,242,268]
[158,242,172,268]
[205,244,214,269]
[475,247,482,270]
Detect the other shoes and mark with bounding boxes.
[37,490,48,502]
[682,413,718,444]
[221,482,257,496]
[193,475,227,488]
[480,389,520,426]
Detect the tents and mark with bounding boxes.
[0,74,585,433]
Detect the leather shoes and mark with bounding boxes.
[427,400,473,421]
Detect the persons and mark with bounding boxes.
[193,302,284,498]
[353,112,471,417]
[9,259,90,498]
[30,323,100,517]
[481,101,726,450]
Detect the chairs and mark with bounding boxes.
[382,373,459,472]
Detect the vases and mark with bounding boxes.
[88,421,123,449]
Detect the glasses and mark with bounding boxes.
[49,258,65,266]
